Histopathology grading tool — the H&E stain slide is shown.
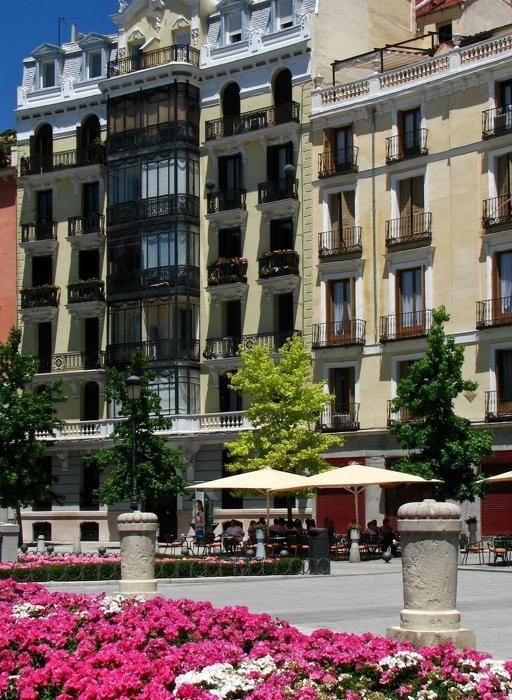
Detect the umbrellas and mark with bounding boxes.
[266,460,447,523]
[476,470,511,483]
[186,467,311,537]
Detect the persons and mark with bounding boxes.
[196,501,203,516]
[221,516,400,560]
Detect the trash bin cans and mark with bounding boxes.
[309,528,330,575]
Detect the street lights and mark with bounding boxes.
[123,375,145,512]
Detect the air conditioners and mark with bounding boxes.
[333,414,350,424]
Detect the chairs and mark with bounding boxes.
[487,537,509,566]
[161,531,385,562]
[462,537,484,565]
